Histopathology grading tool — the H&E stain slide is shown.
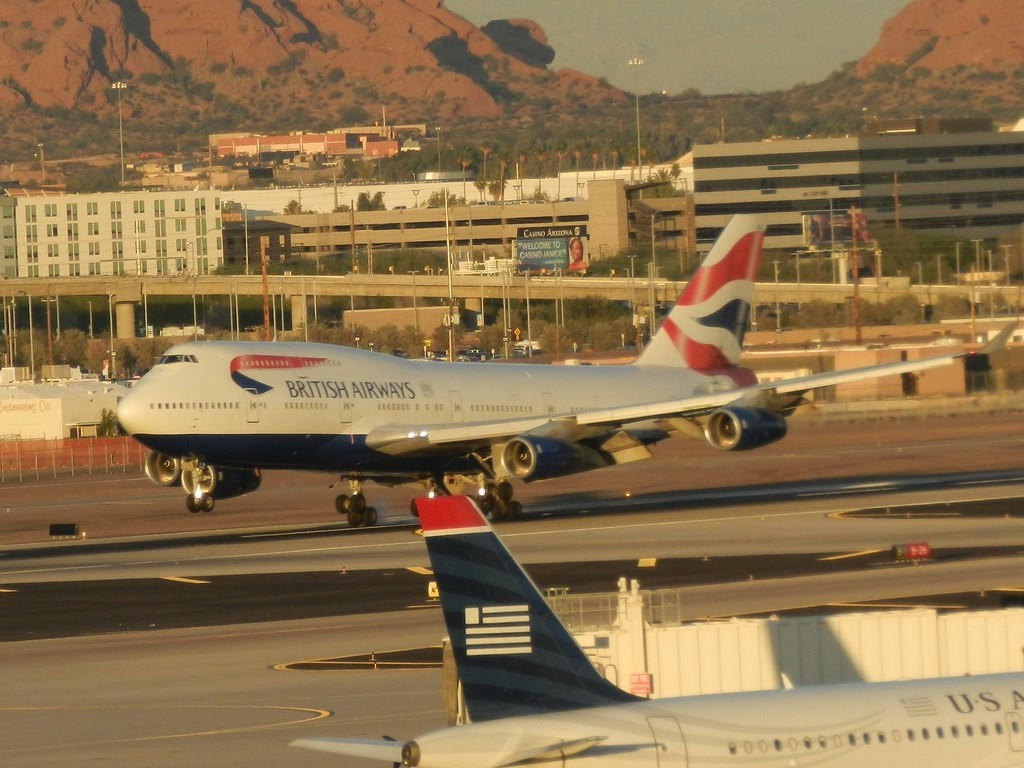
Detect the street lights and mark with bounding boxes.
[628,254,639,301]
[407,270,421,342]
[111,81,127,190]
[190,227,226,341]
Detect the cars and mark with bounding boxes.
[457,339,542,363]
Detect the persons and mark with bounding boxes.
[568,239,587,269]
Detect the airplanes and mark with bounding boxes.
[290,493,1024,768]
[116,213,975,531]
